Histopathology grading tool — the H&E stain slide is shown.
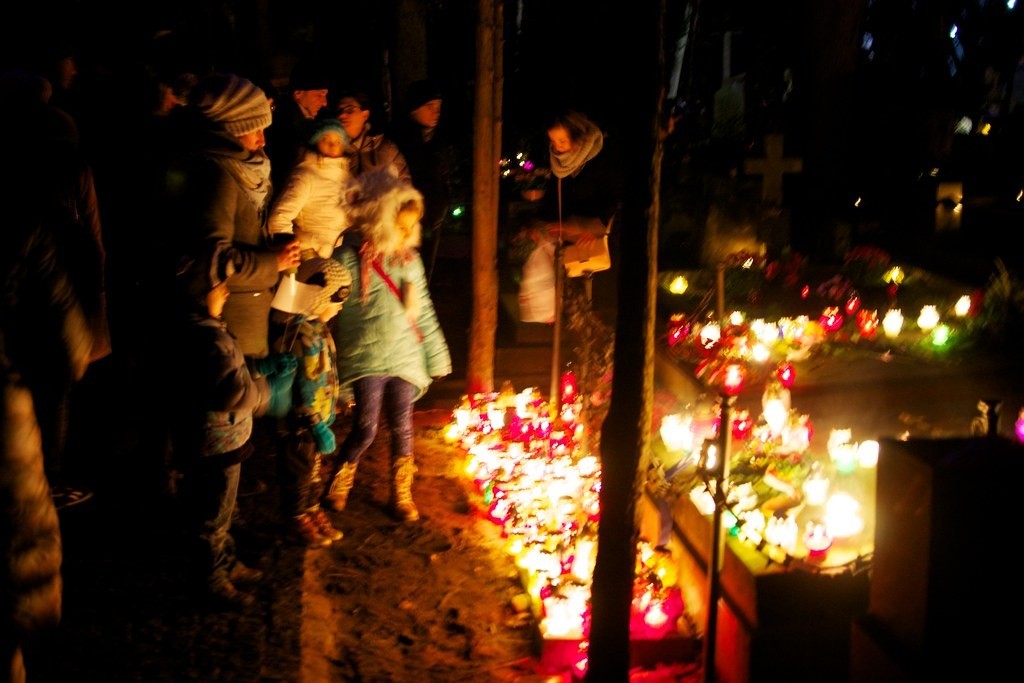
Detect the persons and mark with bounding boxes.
[0,2,641,683]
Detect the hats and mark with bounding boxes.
[196,73,274,137]
[307,105,347,145]
[402,79,443,114]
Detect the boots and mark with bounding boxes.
[390,455,420,522]
[320,461,359,512]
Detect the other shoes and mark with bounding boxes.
[280,514,331,549]
[202,582,255,607]
[305,504,343,542]
[226,561,263,581]
